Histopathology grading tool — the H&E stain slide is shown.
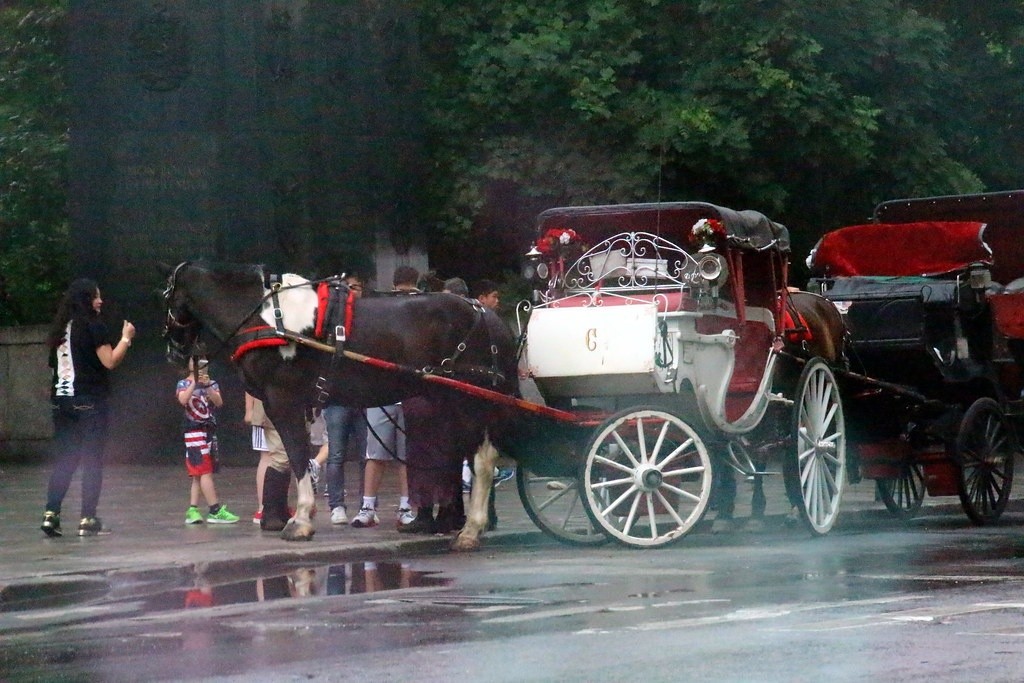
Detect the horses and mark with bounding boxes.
[154,255,545,552]
[710,292,848,534]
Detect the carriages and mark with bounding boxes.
[152,200,848,551]
[706,188,1024,539]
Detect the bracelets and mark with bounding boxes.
[122,337,132,347]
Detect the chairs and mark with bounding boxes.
[847,297,925,352]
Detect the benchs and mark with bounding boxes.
[987,293,1024,340]
[548,291,778,423]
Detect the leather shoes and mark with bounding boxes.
[397,507,434,533]
[432,507,462,532]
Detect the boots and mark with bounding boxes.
[260,466,293,531]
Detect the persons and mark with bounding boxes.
[707,448,722,510]
[441,280,514,494]
[41,278,135,537]
[306,407,349,497]
[244,390,298,524]
[174,354,240,524]
[352,265,419,527]
[324,273,379,522]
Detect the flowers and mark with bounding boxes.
[687,215,725,252]
[537,223,581,261]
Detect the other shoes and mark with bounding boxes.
[253,510,263,524]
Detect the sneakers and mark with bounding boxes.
[462,480,473,494]
[78,516,114,535]
[185,507,203,524]
[307,458,322,494]
[329,506,348,526]
[493,467,515,488]
[393,506,411,527]
[41,511,64,537]
[207,506,240,524]
[350,508,380,528]
[324,484,349,498]
